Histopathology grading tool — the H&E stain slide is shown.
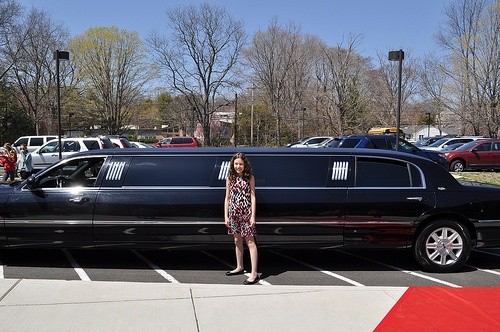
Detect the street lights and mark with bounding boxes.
[388,48,404,151]
[53,50,70,159]
[301,106,307,139]
[425,113,431,137]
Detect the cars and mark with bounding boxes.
[287,127,500,172]
[0,147,500,272]
[0,136,201,179]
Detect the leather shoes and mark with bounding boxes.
[226,268,244,276]
[243,274,260,285]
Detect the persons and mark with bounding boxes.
[0,142,17,182]
[224,153,261,285]
[17,143,33,179]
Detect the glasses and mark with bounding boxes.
[20,148,25,150]
